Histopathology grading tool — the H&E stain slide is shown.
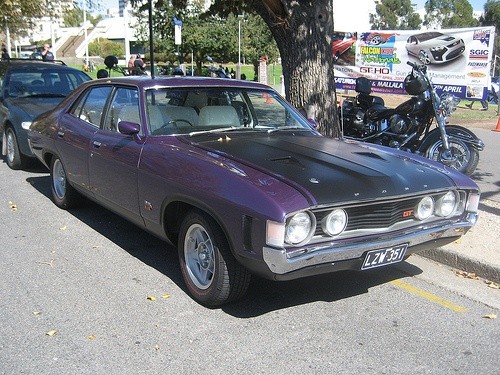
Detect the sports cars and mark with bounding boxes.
[406,32,466,65]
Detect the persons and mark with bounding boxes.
[128,54,148,74]
[40,43,55,63]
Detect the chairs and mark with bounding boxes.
[7,81,24,94]
[51,81,70,93]
[198,105,241,126]
[115,104,164,136]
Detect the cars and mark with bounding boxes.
[28,76,482,309]
[0,62,122,170]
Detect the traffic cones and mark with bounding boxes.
[492,118,500,132]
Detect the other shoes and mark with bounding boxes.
[465,104,472,109]
[479,107,488,111]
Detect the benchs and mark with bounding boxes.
[85,106,199,131]
[17,79,56,93]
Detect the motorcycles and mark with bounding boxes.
[332,61,485,178]
